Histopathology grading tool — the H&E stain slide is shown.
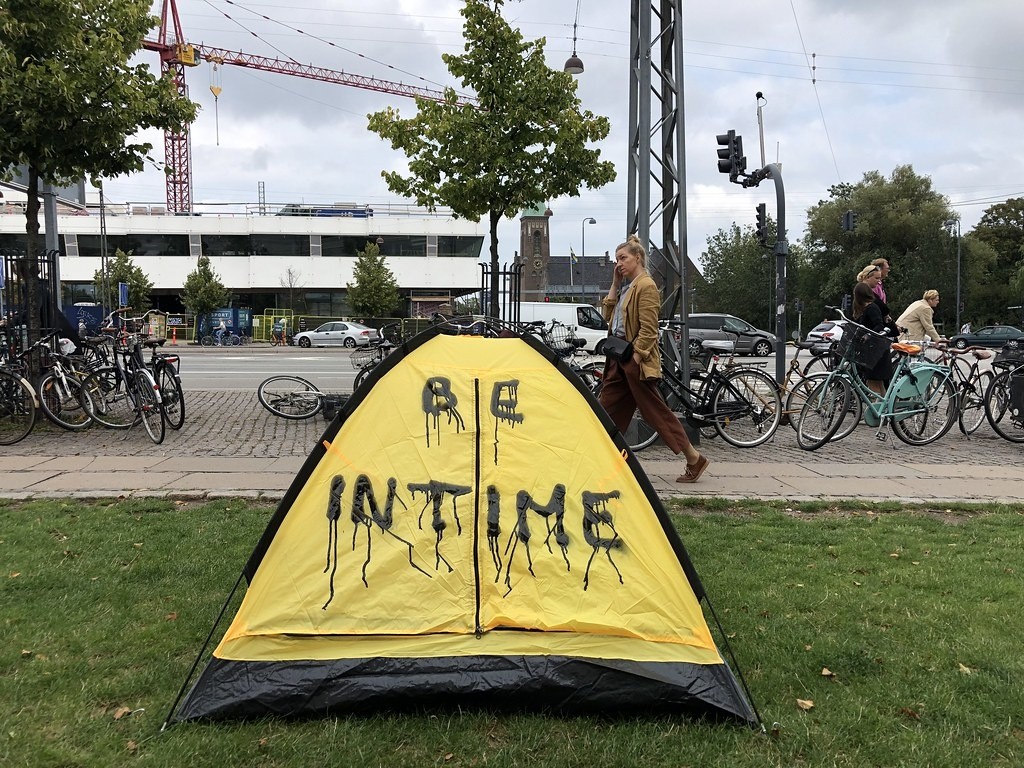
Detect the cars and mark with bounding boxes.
[949,326,1024,350]
[292,321,379,349]
[806,320,848,356]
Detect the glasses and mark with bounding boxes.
[883,267,891,270]
[865,266,880,277]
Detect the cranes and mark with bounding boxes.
[138,0,484,215]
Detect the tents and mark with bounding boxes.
[160,315,767,733]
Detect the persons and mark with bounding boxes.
[599,234,710,482]
[359,319,365,325]
[961,318,1004,335]
[78,319,86,334]
[853,258,901,425]
[887,290,947,349]
[0,310,19,362]
[213,317,226,346]
[103,319,127,350]
[273,318,307,346]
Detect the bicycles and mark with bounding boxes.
[269,331,294,346]
[0,305,185,445]
[201,327,250,347]
[695,325,1024,443]
[349,322,395,392]
[593,320,783,454]
[257,375,350,420]
[798,305,960,451]
[428,312,605,391]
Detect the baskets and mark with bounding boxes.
[835,323,892,370]
[542,325,575,349]
[689,348,713,374]
[349,347,379,370]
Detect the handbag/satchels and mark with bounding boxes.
[603,336,634,364]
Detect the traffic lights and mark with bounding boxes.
[756,203,767,243]
[716,130,746,181]
[847,210,857,231]
[845,294,852,310]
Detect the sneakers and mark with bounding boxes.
[676,453,710,482]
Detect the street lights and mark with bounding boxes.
[582,217,596,303]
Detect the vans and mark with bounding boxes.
[488,302,608,355]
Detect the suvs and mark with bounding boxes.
[674,314,776,357]
